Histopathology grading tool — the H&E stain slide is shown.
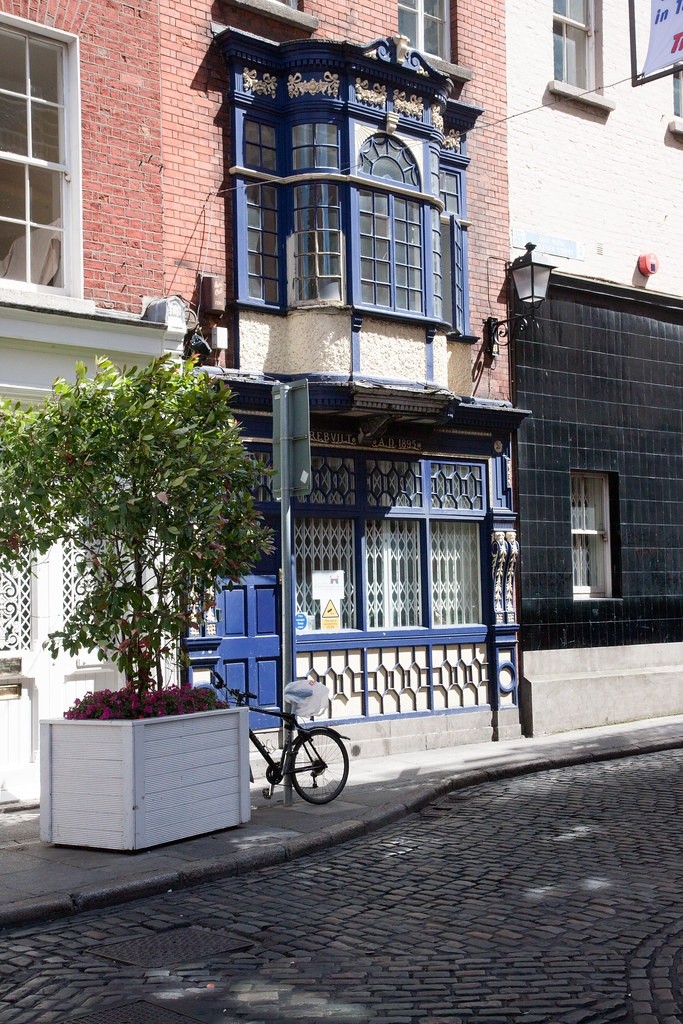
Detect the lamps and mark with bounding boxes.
[484,241,559,358]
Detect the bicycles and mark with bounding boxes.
[210,666,350,805]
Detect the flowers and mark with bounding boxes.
[61,684,229,718]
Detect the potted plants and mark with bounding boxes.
[0,349,286,856]
